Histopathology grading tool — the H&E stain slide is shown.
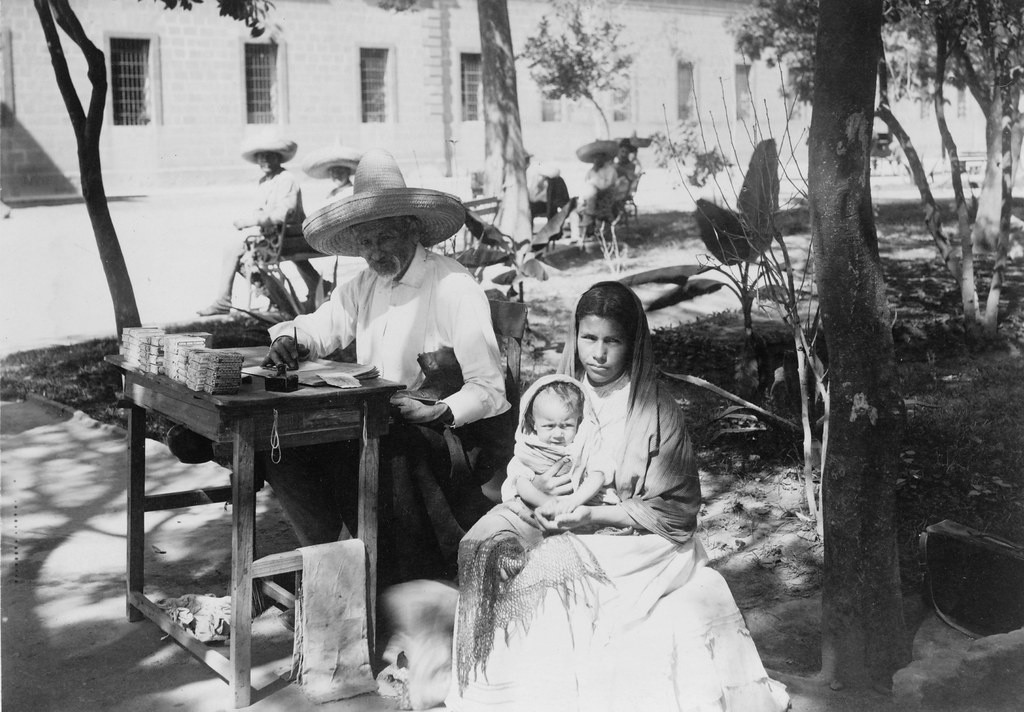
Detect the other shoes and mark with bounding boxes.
[196,305,230,315]
[277,608,295,631]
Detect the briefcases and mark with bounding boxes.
[926,519,1024,628]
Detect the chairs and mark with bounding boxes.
[465,299,529,500]
[441,173,643,289]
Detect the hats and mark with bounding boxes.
[578,139,619,161]
[302,148,467,257]
[240,133,298,163]
[629,132,652,147]
[304,145,364,176]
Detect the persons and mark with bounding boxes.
[218,137,789,712]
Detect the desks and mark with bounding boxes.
[104,346,408,710]
